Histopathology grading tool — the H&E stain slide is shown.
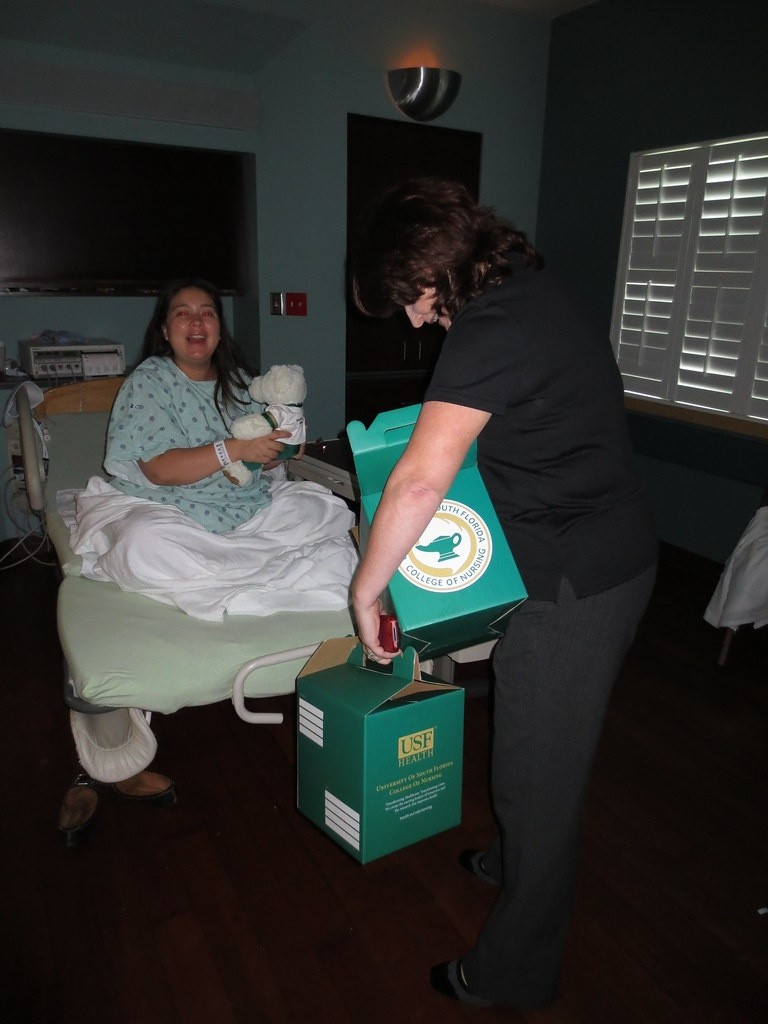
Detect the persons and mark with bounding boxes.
[103,278,326,597]
[351,180,661,1008]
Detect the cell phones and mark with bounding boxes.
[378,615,399,654]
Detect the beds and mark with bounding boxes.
[15,380,457,785]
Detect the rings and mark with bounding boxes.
[368,653,374,660]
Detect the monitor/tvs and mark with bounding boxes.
[0,130,242,299]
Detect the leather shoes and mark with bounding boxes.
[55,769,100,846]
[108,770,178,809]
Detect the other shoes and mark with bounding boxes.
[461,850,501,881]
[423,958,487,1001]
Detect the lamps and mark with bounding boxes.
[388,67,462,122]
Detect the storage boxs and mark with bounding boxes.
[346,400,530,665]
[294,637,466,866]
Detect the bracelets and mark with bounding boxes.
[214,440,231,468]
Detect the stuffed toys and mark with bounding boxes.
[222,364,307,485]
